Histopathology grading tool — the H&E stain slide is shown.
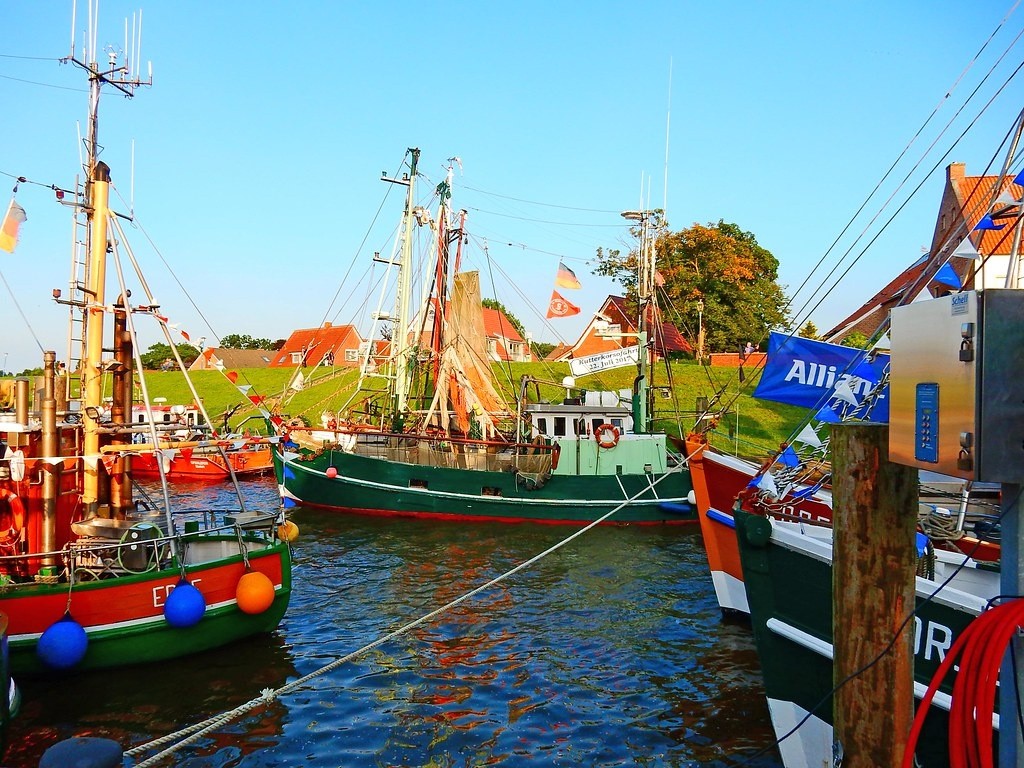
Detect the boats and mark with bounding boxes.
[0,391,276,479]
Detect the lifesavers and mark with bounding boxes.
[595,424,619,449]
[0,488,24,542]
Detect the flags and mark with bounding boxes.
[0,200,26,253]
[547,262,581,319]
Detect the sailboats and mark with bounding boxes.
[0,0,300,681]
[258,146,723,527]
[666,0,1024,768]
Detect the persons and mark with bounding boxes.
[744,342,762,353]
